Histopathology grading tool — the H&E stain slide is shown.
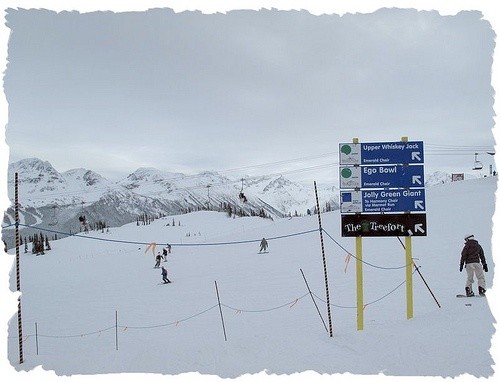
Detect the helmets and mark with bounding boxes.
[464,233,474,240]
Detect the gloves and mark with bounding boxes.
[460,264,463,272]
[483,264,488,272]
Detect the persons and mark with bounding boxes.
[166,244,171,252]
[163,248,167,261]
[161,267,169,284]
[459,233,488,295]
[154,252,164,267]
[260,238,269,252]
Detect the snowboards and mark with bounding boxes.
[457,295,484,297]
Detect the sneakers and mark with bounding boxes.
[479,286,486,294]
[465,287,474,295]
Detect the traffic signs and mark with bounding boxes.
[340,214,428,237]
[338,141,425,165]
[338,165,425,189]
[340,189,426,213]
